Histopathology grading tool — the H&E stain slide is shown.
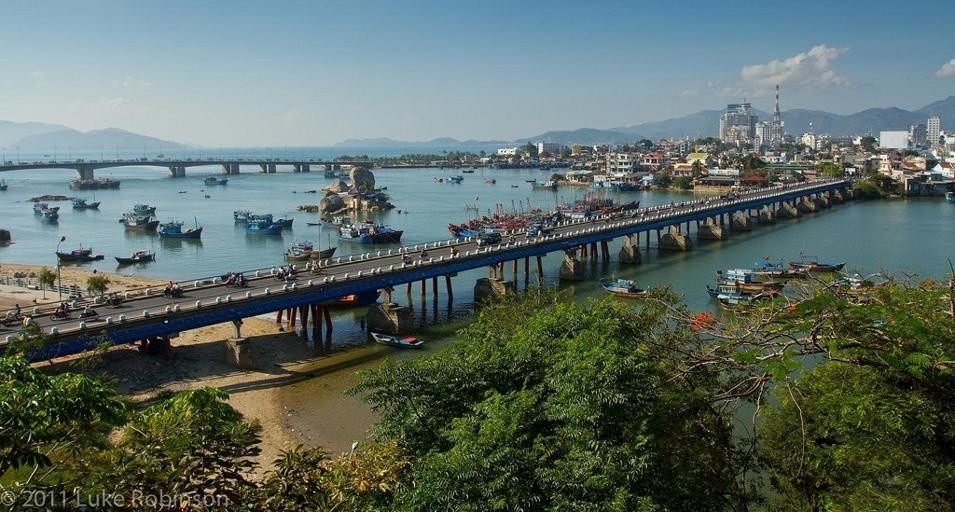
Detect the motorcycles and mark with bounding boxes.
[225,279,249,289]
[162,287,184,299]
[105,298,122,309]
[400,254,430,264]
[273,267,324,282]
[49,311,72,321]
[79,310,99,323]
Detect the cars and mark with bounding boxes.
[476,233,502,246]
[720,192,735,201]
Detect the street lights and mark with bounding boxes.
[56,237,65,300]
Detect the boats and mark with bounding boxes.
[204,177,228,186]
[370,332,425,349]
[705,247,848,315]
[945,192,954,203]
[0,176,210,265]
[232,207,404,263]
[433,168,536,189]
[599,278,647,299]
[447,183,641,241]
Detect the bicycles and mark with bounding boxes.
[17,323,44,335]
